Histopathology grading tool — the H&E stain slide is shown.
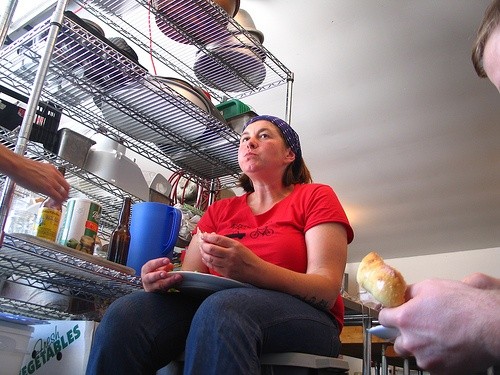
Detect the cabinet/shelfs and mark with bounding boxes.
[0,0,294,322]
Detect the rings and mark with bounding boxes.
[59,187,66,195]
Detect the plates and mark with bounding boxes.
[168,270,248,291]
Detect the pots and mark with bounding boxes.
[18,0,267,177]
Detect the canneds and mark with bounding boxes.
[56,196,102,259]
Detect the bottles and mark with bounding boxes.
[107,196,131,266]
[35,165,68,244]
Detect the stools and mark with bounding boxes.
[256,352,350,375]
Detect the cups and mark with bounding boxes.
[55,198,103,256]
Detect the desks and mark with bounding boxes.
[339,287,427,375]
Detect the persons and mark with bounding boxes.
[87,115,354,375]
[0,144,70,208]
[378,0,500,375]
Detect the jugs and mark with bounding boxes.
[126,204,182,276]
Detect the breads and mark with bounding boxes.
[357,249,410,309]
[196,227,216,249]
